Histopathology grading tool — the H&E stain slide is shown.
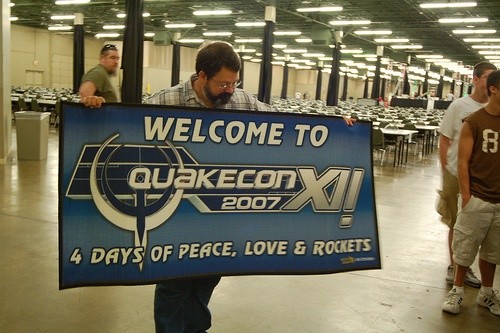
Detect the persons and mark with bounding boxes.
[441,69,500,316]
[138,40,356,333]
[79,44,120,108]
[436,62,498,288]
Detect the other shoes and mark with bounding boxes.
[464,267,482,287]
[445,265,455,284]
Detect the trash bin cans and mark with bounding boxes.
[15,111,51,162]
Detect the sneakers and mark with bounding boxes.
[440,285,466,316]
[476,287,500,315]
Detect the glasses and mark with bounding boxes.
[106,44,119,50]
[208,78,242,89]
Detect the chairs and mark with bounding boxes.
[18,97,61,127]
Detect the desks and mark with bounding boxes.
[11,97,57,105]
[397,124,440,154]
[381,128,418,165]
